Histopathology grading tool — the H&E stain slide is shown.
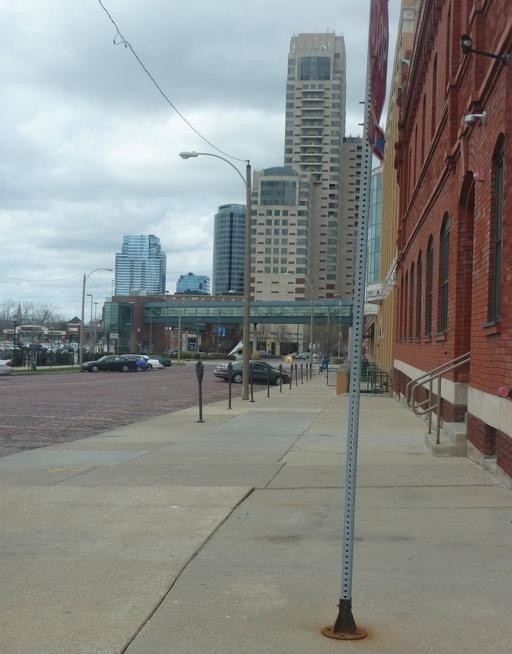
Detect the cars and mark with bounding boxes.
[213,360,290,386]
[0,359,13,375]
[81,354,171,373]
[0,341,79,355]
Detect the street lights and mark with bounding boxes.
[87,294,93,362]
[93,302,99,361]
[79,267,114,372]
[179,151,251,400]
[13,320,17,367]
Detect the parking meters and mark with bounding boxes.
[289,363,312,388]
[195,360,282,424]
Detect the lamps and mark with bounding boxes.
[465,109,486,125]
[458,33,512,66]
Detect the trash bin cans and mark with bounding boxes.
[336,370,350,394]
[320,354,328,371]
